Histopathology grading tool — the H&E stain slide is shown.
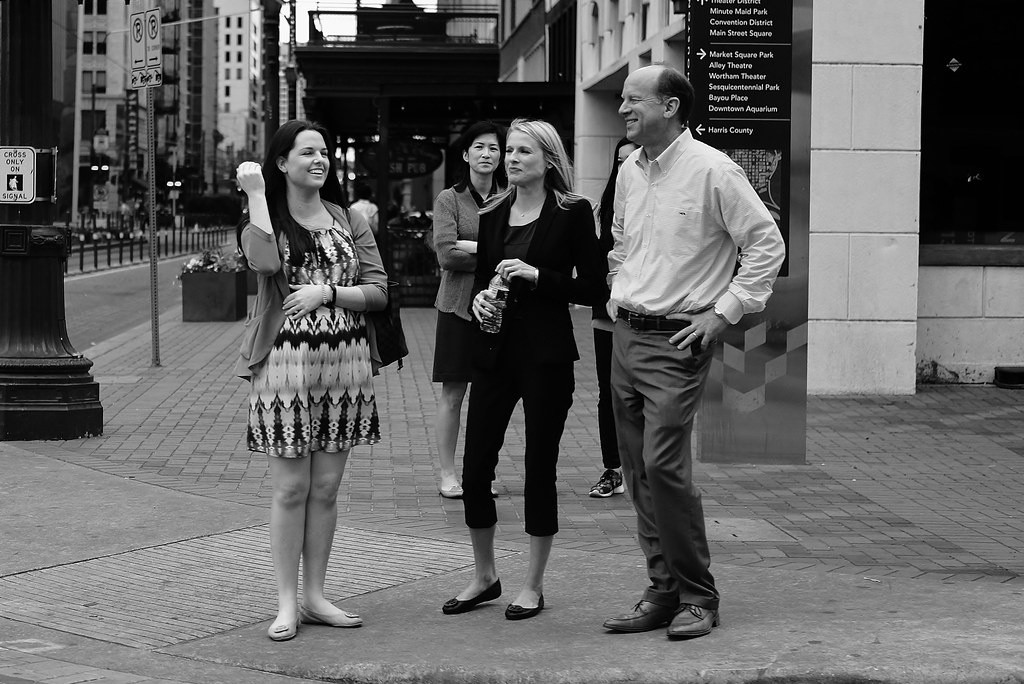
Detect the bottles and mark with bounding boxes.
[480,274,510,333]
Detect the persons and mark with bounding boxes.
[236,120,389,641]
[603,66,785,636]
[349,184,378,232]
[120,198,132,228]
[589,138,641,497]
[134,201,150,235]
[442,121,604,619]
[432,122,506,497]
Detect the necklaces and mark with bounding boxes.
[521,200,545,217]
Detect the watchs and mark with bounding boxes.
[714,307,730,326]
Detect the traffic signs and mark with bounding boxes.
[145,8,165,87]
[130,11,147,91]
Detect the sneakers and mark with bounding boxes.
[589,468,624,497]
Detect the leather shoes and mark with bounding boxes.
[603,600,673,632]
[666,602,720,635]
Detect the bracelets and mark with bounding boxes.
[321,284,327,304]
[329,285,336,305]
[535,269,538,284]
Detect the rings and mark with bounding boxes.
[693,333,698,338]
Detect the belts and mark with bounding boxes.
[616,307,692,332]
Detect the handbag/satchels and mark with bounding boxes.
[367,281,409,367]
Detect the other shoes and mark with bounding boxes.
[442,577,502,614]
[298,604,364,626]
[268,614,301,641]
[491,486,499,496]
[505,593,544,620]
[438,479,464,498]
